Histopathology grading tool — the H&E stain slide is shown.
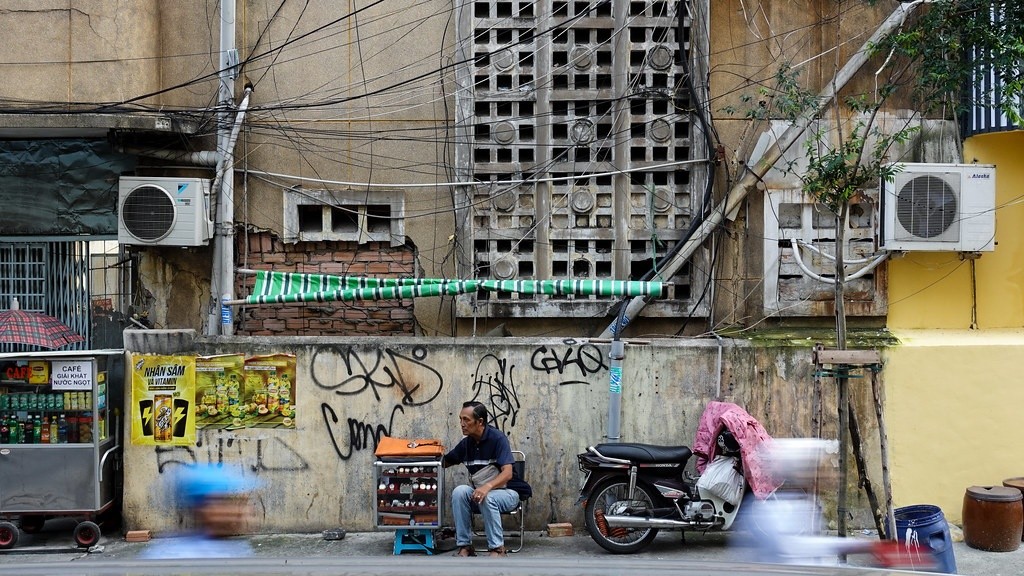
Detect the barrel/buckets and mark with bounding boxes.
[885,504,957,573]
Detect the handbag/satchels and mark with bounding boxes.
[469,465,507,488]
[696,456,744,505]
[465,461,532,501]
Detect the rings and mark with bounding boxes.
[477,496,480,499]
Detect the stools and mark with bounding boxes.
[392,529,437,556]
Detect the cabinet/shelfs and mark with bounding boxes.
[372,455,446,531]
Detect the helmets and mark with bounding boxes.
[181,465,261,514]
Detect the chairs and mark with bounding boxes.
[456,451,526,553]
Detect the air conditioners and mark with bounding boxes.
[877,163,996,252]
[118,175,215,249]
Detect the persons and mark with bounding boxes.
[267,371,279,399]
[279,374,290,404]
[442,401,519,557]
[228,375,239,405]
[216,372,227,393]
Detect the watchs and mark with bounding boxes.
[401,500,404,507]
[431,499,436,506]
[399,468,410,473]
[379,481,386,490]
[412,467,437,473]
[389,479,395,490]
[413,482,437,490]
[405,500,425,507]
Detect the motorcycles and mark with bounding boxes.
[573,400,817,555]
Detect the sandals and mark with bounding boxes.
[452,545,477,557]
[487,548,508,559]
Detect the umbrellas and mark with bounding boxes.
[0,309,85,349]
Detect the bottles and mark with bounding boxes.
[203,371,291,417]
[11,298,19,311]
[0,414,102,444]
